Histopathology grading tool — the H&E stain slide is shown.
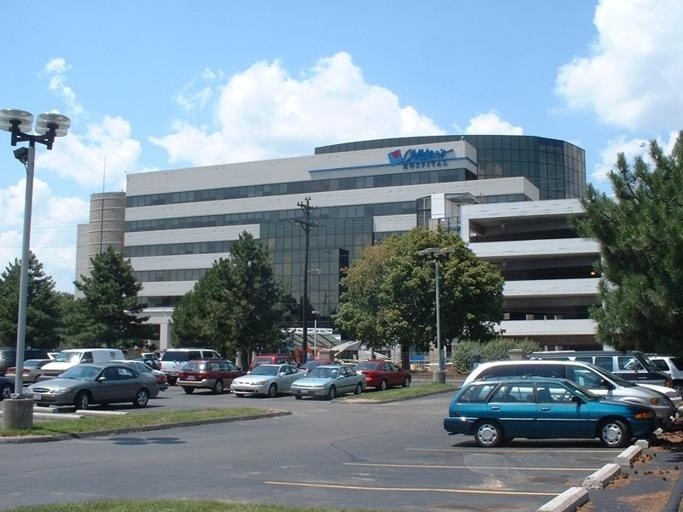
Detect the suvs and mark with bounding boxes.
[443,342,683,448]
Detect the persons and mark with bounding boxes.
[401,354,410,387]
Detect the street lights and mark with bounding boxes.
[0,104,72,400]
[311,310,320,351]
[416,244,447,378]
